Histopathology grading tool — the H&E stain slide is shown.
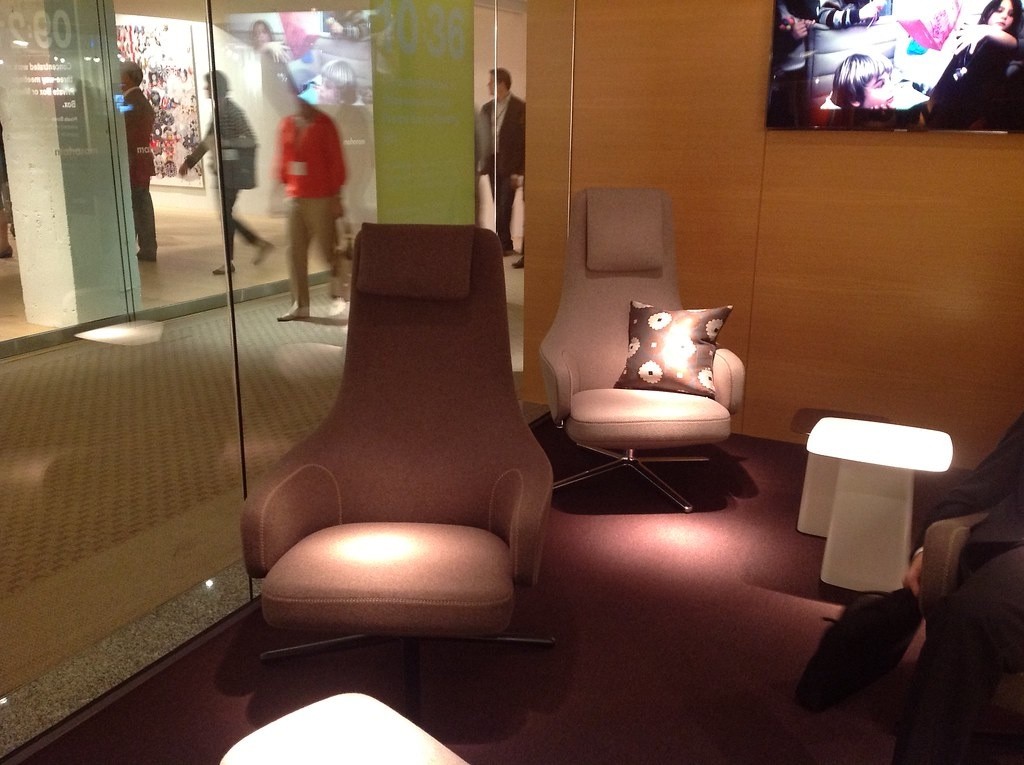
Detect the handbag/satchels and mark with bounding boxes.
[226,151,253,190]
[794,587,924,713]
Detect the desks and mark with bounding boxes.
[219,693,470,765]
[795,417,955,596]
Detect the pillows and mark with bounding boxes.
[612,300,734,399]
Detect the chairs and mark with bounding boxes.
[239,222,557,724]
[535,186,745,513]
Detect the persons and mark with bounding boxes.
[771,0,821,104]
[267,71,350,320]
[326,11,369,40]
[477,67,526,252]
[319,60,365,105]
[250,20,291,63]
[121,61,158,262]
[818,0,884,27]
[0,195,13,258]
[929,0,1024,128]
[177,70,274,274]
[821,51,894,110]
[892,412,1024,765]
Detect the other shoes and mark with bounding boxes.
[254,241,272,269]
[503,249,514,257]
[213,265,236,276]
[137,251,157,262]
[0,246,12,259]
[511,256,524,268]
[277,308,309,321]
